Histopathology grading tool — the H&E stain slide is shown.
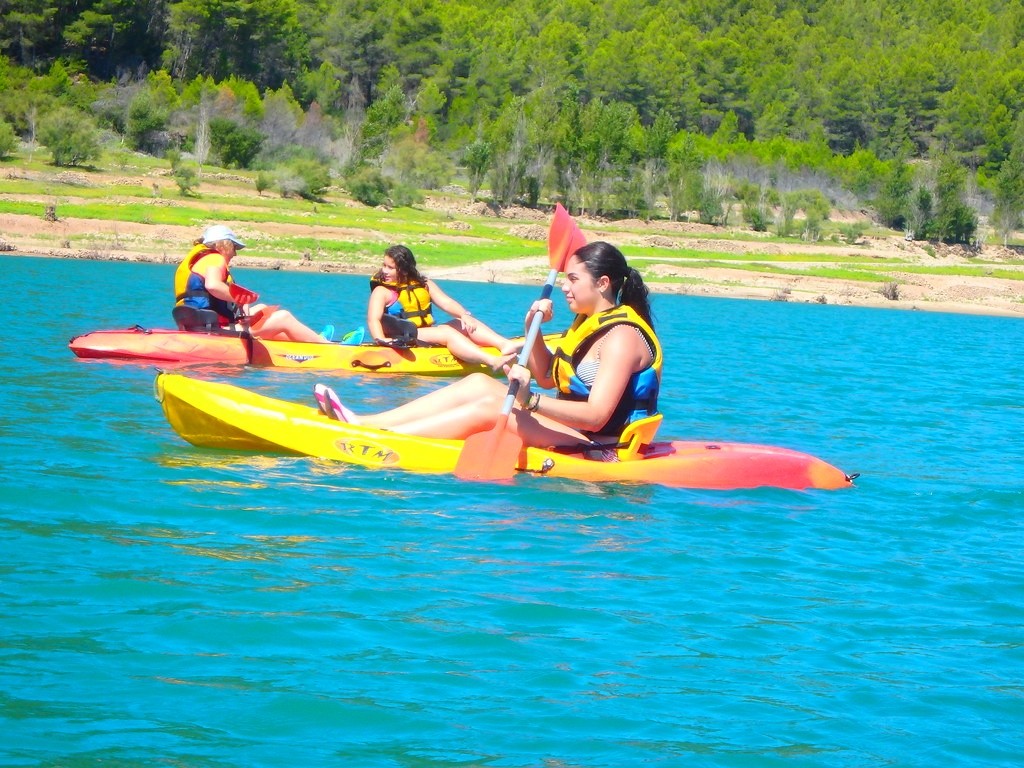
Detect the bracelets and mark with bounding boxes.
[460,310,472,317]
[524,392,541,413]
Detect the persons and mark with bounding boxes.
[368,244,526,372]
[174,224,364,345]
[314,242,661,448]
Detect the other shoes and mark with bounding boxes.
[324,389,348,422]
[313,384,327,415]
[321,325,334,341]
[340,326,364,345]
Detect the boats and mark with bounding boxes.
[154,367,856,492]
[68,328,565,377]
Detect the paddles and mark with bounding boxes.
[228,282,280,329]
[456,201,587,482]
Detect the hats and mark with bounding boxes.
[202,225,245,250]
